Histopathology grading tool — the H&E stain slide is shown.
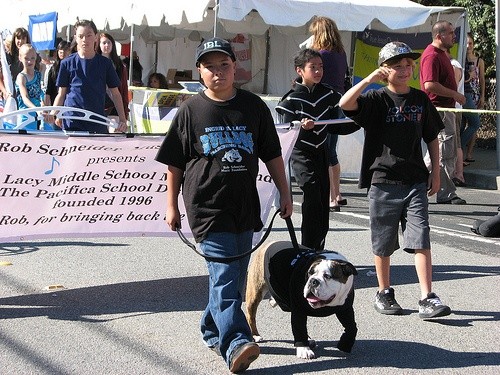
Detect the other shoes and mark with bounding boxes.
[452,176,466,187]
[337,198,348,205]
[329,205,341,212]
[437,196,467,205]
[229,342,261,373]
[209,341,223,355]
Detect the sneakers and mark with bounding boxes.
[374,287,404,315]
[418,292,451,319]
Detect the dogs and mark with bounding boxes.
[245,239,358,358]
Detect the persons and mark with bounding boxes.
[0,27,43,99]
[309,17,348,211]
[148,73,169,90]
[419,20,468,204]
[44,32,144,129]
[15,44,52,130]
[460,32,485,166]
[154,38,293,372]
[338,40,450,318]
[275,49,361,250]
[43,21,127,131]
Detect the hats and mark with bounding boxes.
[377,41,421,67]
[195,37,236,67]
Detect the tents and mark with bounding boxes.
[0,0,470,180]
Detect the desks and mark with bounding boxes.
[123,104,181,134]
